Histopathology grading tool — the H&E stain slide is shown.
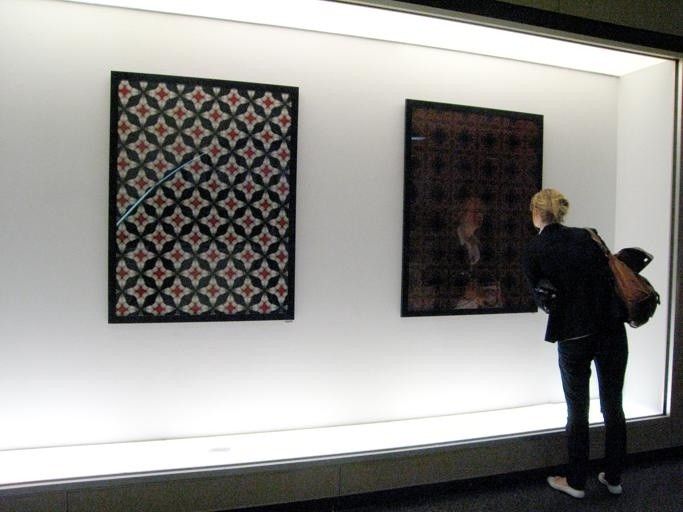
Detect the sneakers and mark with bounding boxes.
[598,471,623,495]
[546,475,586,500]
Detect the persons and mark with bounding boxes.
[422,195,491,308]
[518,186,630,499]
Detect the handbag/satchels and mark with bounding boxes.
[584,227,660,328]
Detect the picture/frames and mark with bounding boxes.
[400,98,544,318]
[105,71,297,323]
